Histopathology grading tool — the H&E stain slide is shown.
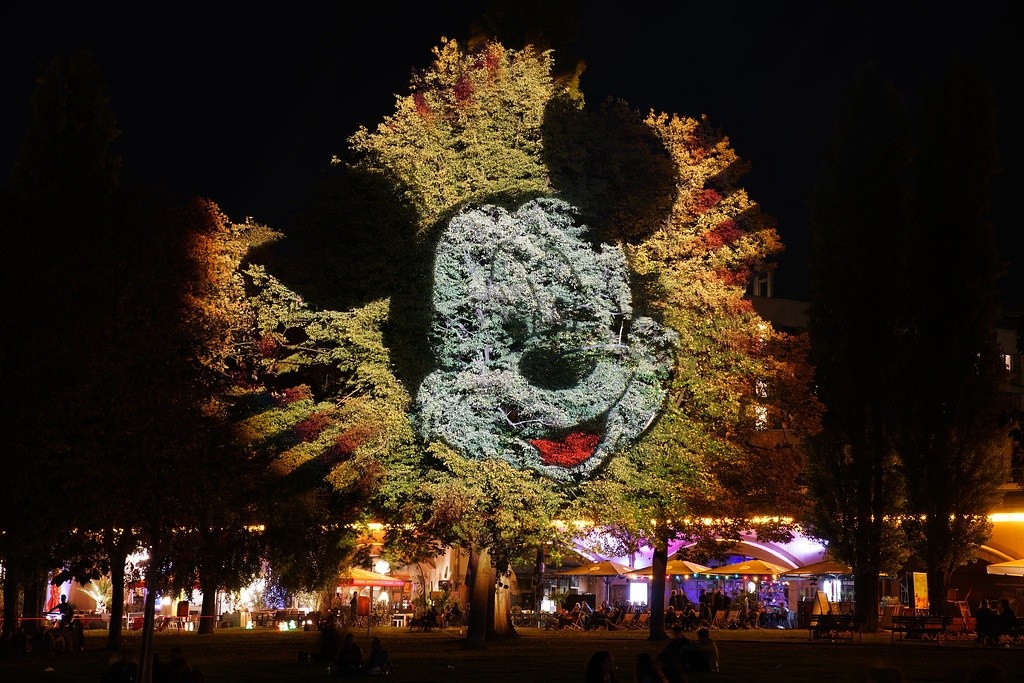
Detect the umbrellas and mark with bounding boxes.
[552,559,636,600]
[322,564,405,589]
[702,557,790,590]
[784,555,890,591]
[633,559,712,590]
[985,558,1024,583]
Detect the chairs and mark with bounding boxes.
[511,602,796,632]
[250,608,316,629]
[127,618,144,636]
[153,617,172,636]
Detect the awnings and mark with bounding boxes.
[125,572,228,631]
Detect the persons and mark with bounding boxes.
[349,592,361,626]
[976,598,1022,642]
[442,602,472,626]
[46,594,73,627]
[542,584,796,631]
[332,592,343,613]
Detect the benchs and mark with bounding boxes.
[809,613,869,646]
[408,613,447,631]
[973,616,1024,647]
[891,615,954,647]
